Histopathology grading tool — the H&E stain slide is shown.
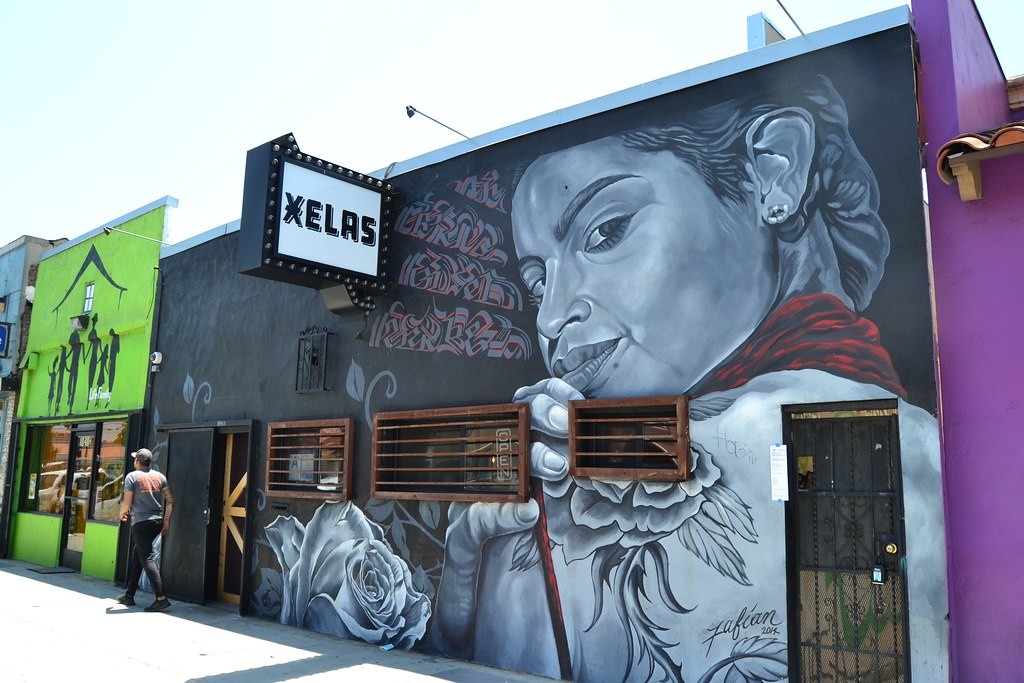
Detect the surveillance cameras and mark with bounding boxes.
[150,352,162,364]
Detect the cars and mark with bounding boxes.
[35,459,125,522]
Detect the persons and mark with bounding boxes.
[118,448,172,610]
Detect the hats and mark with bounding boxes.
[130,449,153,465]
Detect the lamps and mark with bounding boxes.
[103,226,171,246]
[406,105,471,140]
[70,315,90,331]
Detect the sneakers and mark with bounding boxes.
[144,598,171,612]
[119,596,135,606]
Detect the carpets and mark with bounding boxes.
[26,567,76,575]
